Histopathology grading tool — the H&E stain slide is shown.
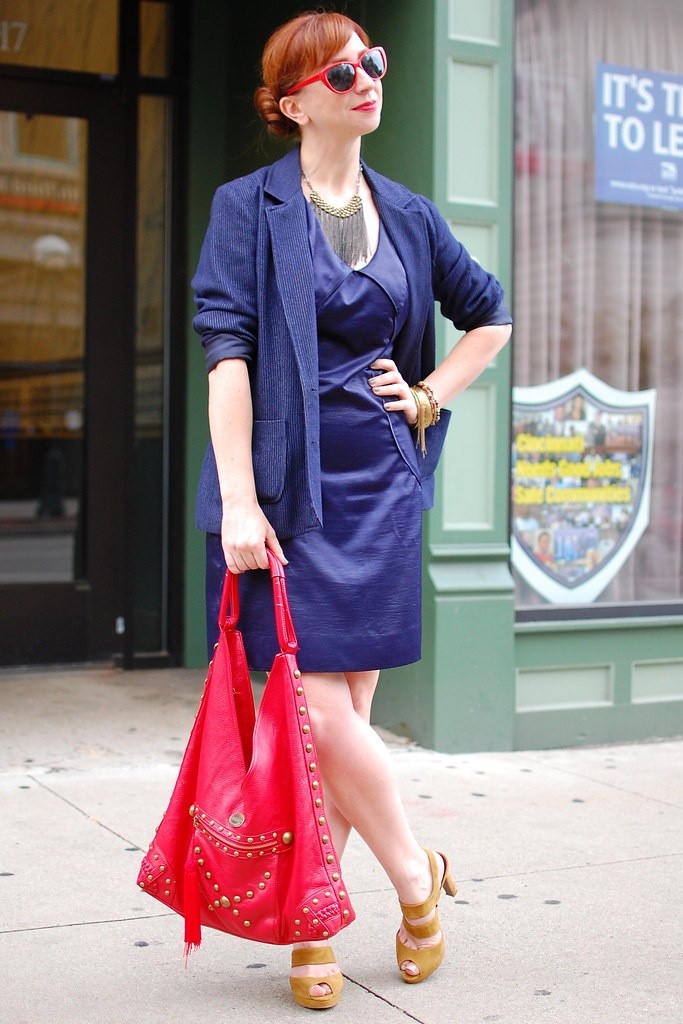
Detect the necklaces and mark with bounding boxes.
[299,164,374,269]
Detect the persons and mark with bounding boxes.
[188,12,514,1008]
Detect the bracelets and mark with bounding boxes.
[409,384,434,458]
[415,380,441,427]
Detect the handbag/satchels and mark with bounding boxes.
[137,547,356,942]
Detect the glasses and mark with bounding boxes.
[284,46,388,94]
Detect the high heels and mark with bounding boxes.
[290,945,346,1011]
[396,849,459,985]
